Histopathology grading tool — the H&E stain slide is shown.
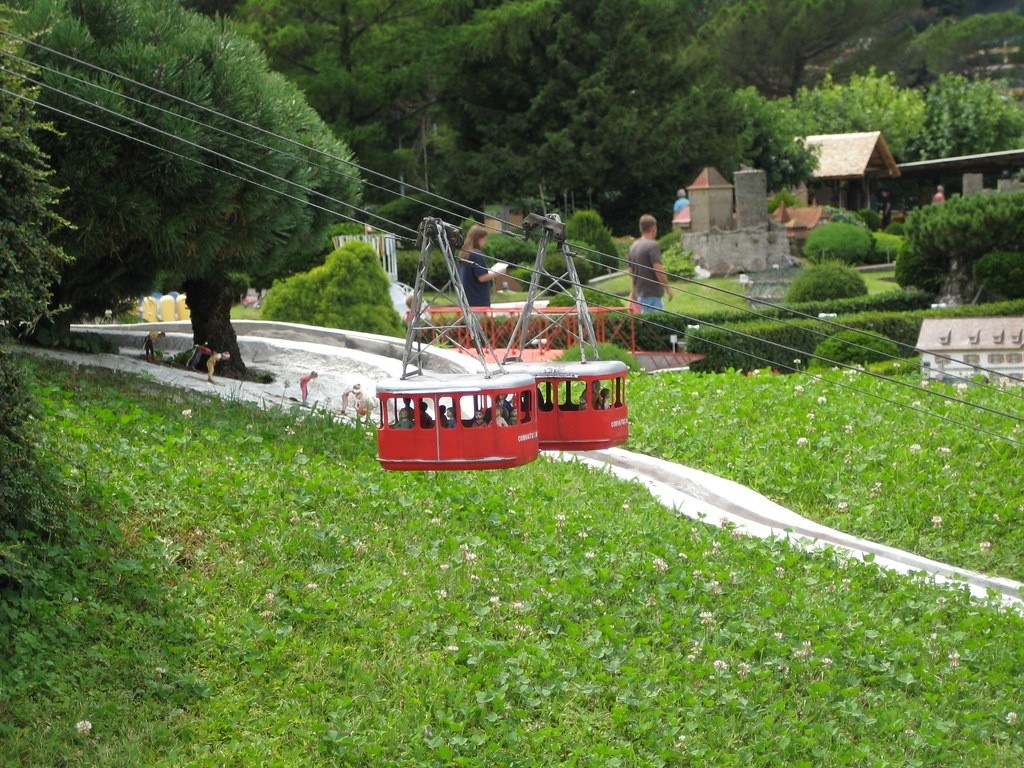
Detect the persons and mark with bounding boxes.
[207,351,230,382]
[145,331,165,361]
[396,388,544,429]
[341,384,373,416]
[458,227,505,335]
[300,371,319,405]
[628,214,673,313]
[404,294,430,338]
[931,186,945,204]
[191,344,217,370]
[876,190,893,227]
[673,189,688,215]
[579,381,613,411]
